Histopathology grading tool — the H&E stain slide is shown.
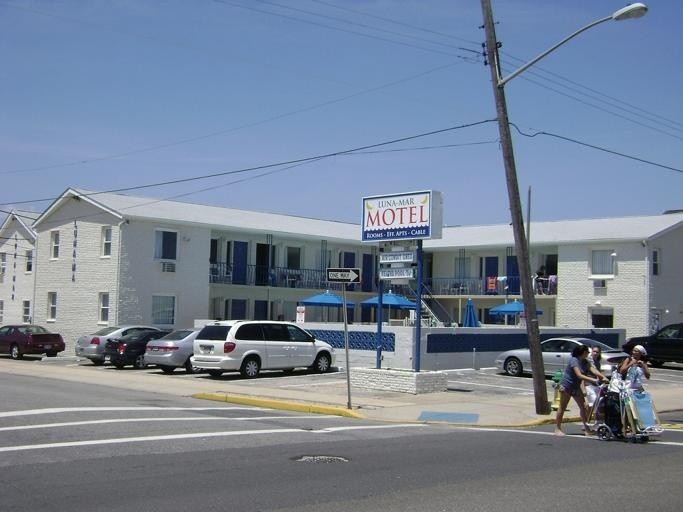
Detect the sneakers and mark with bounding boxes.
[554,429,567,437]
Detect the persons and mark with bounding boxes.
[533,265,544,295]
[581,344,610,432]
[554,344,604,437]
[618,343,663,437]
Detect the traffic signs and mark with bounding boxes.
[326,267,361,284]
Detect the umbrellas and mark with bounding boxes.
[359,289,421,325]
[462,296,480,326]
[295,289,356,323]
[488,298,544,325]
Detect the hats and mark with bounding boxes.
[633,344,648,356]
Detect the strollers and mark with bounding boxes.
[585,369,661,444]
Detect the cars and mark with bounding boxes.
[0,324,66,360]
[74,324,202,375]
[495,337,631,377]
[621,323,683,366]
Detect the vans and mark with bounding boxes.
[190,319,337,379]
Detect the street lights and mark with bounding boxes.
[479,1,649,416]
